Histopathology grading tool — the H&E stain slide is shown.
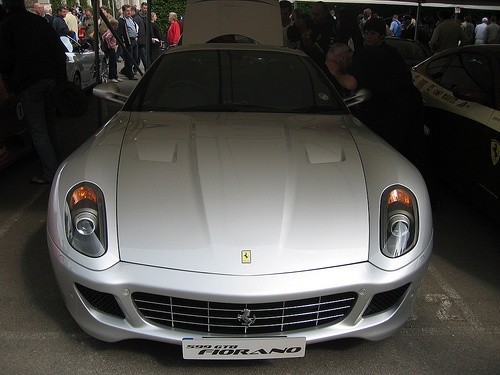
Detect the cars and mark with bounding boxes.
[412,44,500,204]
[61,34,111,92]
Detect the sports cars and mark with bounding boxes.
[46,0,434,359]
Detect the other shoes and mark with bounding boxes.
[31,175,52,184]
[108,78,118,83]
[129,75,138,80]
[116,78,123,82]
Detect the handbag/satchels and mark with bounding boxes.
[101,38,108,52]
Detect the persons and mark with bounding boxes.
[32,2,185,82]
[0,0,68,184]
[281,1,500,164]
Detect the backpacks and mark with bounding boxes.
[392,20,401,37]
